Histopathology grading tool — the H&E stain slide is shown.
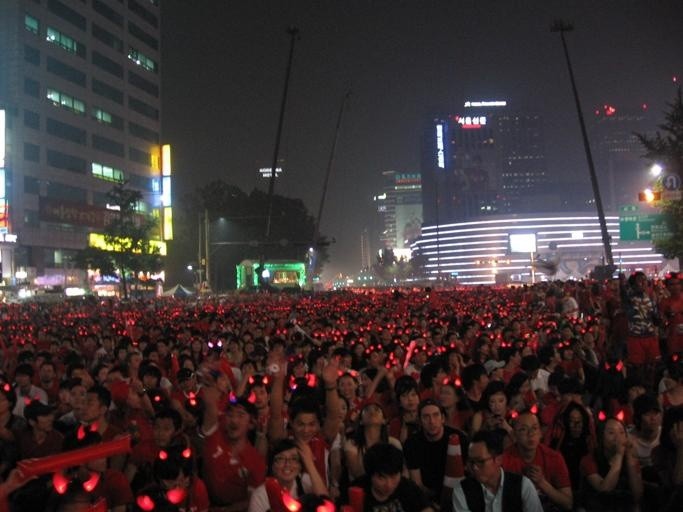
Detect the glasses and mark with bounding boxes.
[269,417,656,482]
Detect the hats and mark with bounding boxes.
[484,359,505,375]
[23,340,332,419]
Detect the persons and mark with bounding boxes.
[1,273,681,509]
